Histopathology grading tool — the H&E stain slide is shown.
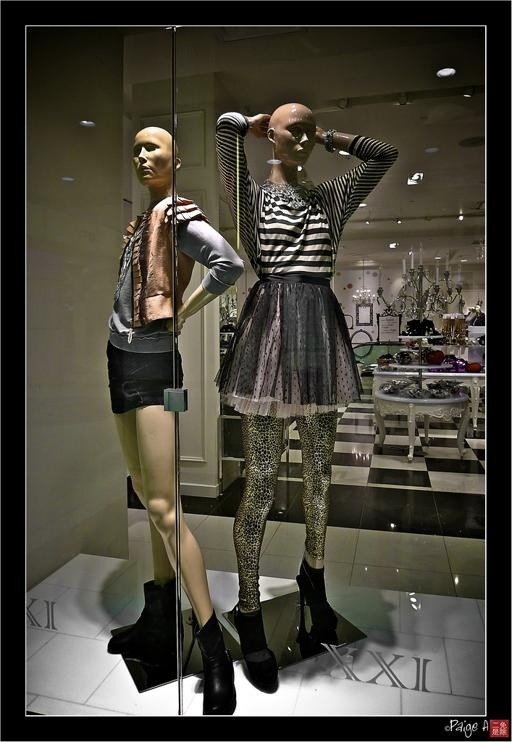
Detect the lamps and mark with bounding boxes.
[352,259,376,308]
[338,86,474,110]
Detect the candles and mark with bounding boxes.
[402,242,462,289]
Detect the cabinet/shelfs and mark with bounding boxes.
[374,334,470,404]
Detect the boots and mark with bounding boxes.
[296,557,338,631]
[193,607,235,715]
[108,576,182,654]
[236,603,278,691]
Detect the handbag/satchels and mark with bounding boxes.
[378,375,471,398]
[407,320,439,335]
[377,347,482,372]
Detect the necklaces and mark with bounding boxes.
[262,179,311,210]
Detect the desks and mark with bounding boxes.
[369,396,470,462]
[372,367,485,436]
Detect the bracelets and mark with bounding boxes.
[324,128,337,153]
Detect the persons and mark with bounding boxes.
[106,126,244,716]
[215,101,399,694]
